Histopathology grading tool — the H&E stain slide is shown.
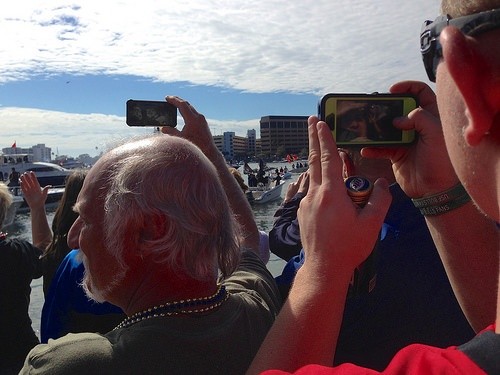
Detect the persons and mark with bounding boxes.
[18,96,283,375]
[230,0,500,375]
[0,166,126,375]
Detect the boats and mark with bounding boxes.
[245,179,286,204]
[228,160,292,180]
[0,153,72,213]
[289,165,309,174]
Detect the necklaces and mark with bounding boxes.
[114,284,231,331]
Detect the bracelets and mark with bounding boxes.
[412,182,466,209]
[420,192,471,216]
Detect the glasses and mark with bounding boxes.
[420,9,500,83]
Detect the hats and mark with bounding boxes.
[275,247,377,301]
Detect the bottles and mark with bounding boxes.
[346,176,380,303]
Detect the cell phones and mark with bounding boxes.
[126,99,177,126]
[319,93,419,147]
